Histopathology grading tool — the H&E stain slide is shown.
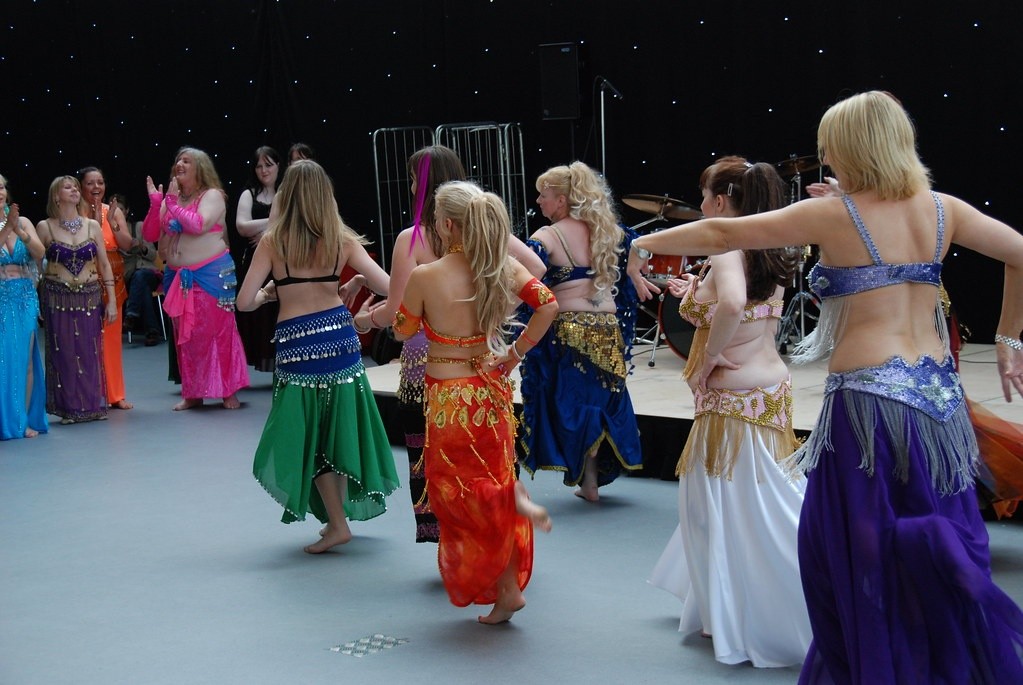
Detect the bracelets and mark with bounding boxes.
[520,329,537,345]
[370,310,383,329]
[705,344,718,357]
[104,283,115,286]
[111,224,120,231]
[994,334,1023,351]
[510,340,526,362]
[260,288,269,304]
[104,279,116,282]
[353,317,371,334]
[20,233,31,244]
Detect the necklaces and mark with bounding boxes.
[0,216,7,231]
[263,189,272,213]
[443,243,464,254]
[57,215,83,234]
[180,185,203,207]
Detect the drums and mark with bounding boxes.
[658,260,712,362]
[645,228,704,288]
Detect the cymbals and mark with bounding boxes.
[775,155,819,176]
[621,194,704,220]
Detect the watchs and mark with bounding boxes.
[631,240,649,259]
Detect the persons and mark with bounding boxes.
[235,160,402,553]
[354,146,643,624]
[805,176,845,197]
[627,89,1023,685]
[0,143,312,441]
